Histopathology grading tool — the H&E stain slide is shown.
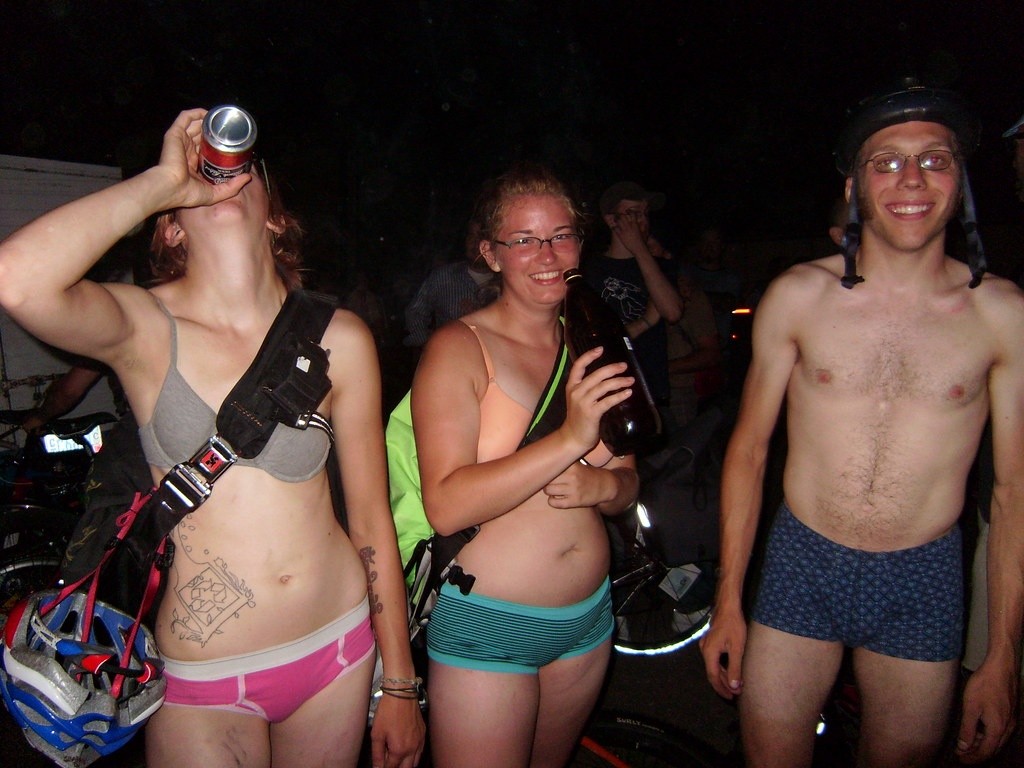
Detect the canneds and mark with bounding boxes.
[197,104,256,184]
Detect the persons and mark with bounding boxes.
[1,106,433,768]
[379,162,641,768]
[22,245,168,635]
[693,86,1024,766]
[292,112,1024,433]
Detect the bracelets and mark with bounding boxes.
[378,677,429,712]
[642,315,651,328]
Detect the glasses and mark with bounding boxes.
[616,206,654,219]
[861,149,955,172]
[493,226,585,255]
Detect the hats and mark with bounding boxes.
[1002,114,1024,137]
[834,72,970,167]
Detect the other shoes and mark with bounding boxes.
[671,607,710,632]
[616,616,630,642]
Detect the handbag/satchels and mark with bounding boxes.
[383,383,455,623]
[60,409,158,615]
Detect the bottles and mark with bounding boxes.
[563,269,662,457]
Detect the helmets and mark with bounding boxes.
[0,590,166,767]
